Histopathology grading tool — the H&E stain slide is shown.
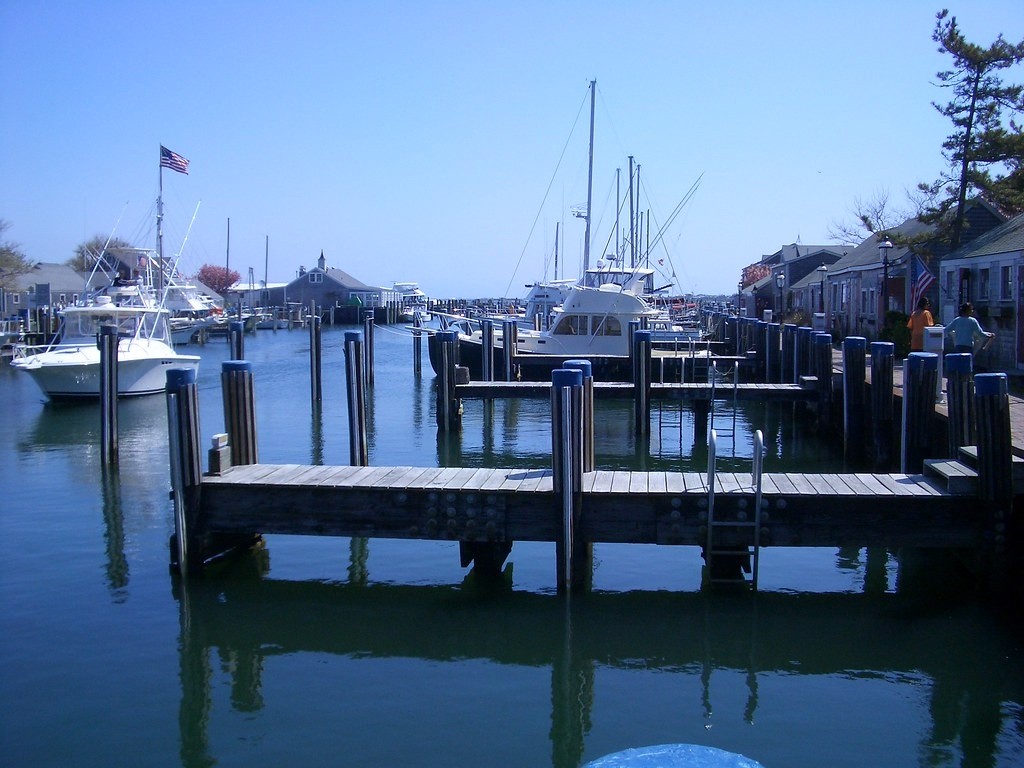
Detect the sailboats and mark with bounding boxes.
[415,78,747,397]
[122,216,288,344]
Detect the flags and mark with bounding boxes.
[911,256,936,312]
[160,145,190,175]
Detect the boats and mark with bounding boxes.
[8,199,203,407]
[379,282,431,322]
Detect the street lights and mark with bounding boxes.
[777,273,786,331]
[817,263,828,312]
[737,281,742,317]
[753,284,758,318]
[878,235,893,341]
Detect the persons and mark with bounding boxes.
[907,297,934,352]
[113,268,143,287]
[944,302,994,354]
[231,303,238,315]
